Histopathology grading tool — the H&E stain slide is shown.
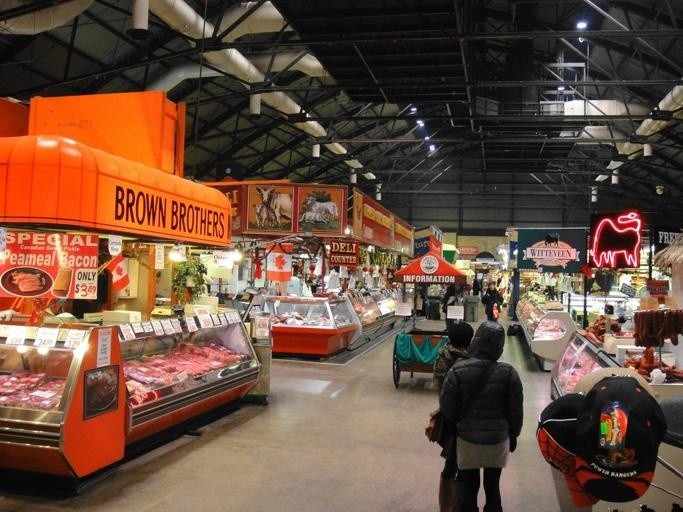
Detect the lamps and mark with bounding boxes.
[312,143,382,201]
[130,0,150,32]
[590,141,652,205]
[249,93,261,117]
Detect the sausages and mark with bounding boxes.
[587,319,621,342]
[623,308,683,383]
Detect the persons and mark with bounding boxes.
[431,322,475,512]
[437,321,524,512]
[481,280,503,323]
[355,280,364,291]
[286,264,312,296]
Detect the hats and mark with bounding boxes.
[537,368,683,507]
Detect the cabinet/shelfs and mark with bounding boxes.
[549,329,683,399]
[263,290,400,361]
[514,294,577,372]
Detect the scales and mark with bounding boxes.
[150,305,176,317]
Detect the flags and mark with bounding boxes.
[265,242,292,282]
[105,252,130,292]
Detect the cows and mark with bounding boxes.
[251,204,270,227]
[255,185,292,229]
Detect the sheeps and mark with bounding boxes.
[305,196,339,220]
[299,211,330,225]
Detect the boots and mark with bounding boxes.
[447,480,456,512]
[438,473,455,512]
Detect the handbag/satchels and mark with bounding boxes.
[424,410,444,443]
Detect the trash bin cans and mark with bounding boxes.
[465,296,478,322]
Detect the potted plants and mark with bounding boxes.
[170,258,214,307]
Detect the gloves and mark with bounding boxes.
[509,437,516,452]
